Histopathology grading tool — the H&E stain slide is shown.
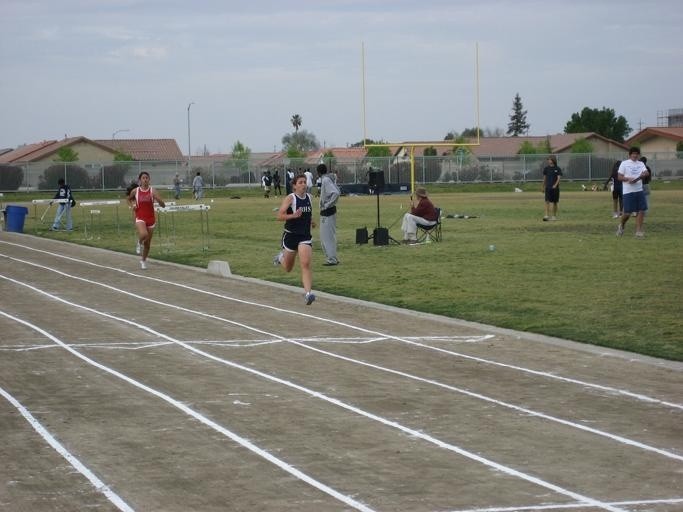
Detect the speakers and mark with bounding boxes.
[356,228,368,244]
[373,228,389,245]
[368,167,384,192]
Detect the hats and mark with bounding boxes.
[415,188,427,197]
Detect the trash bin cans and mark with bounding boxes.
[5,205,28,232]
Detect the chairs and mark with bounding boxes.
[415,207,443,245]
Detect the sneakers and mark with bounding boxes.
[613,211,645,240]
[543,214,560,221]
[323,260,338,266]
[305,294,314,305]
[136,241,147,269]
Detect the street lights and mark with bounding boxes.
[112,128,132,139]
[187,101,193,186]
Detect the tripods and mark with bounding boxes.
[361,191,401,244]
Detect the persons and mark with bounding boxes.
[400,186,438,245]
[126,179,139,209]
[261,168,337,199]
[317,164,342,265]
[366,166,375,195]
[173,173,183,199]
[48,177,74,232]
[541,155,563,221]
[602,146,651,238]
[192,172,204,200]
[129,171,165,270]
[273,173,316,306]
